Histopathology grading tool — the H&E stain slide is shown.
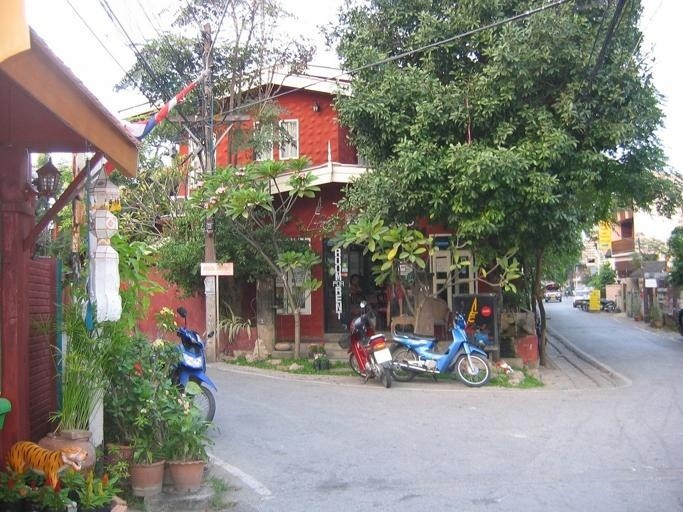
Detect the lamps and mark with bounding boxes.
[311,100,319,112]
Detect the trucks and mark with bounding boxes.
[544,283,562,302]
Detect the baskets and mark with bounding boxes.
[338,335,351,349]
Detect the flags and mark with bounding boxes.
[104,81,196,176]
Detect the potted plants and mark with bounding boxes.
[0,292,219,511]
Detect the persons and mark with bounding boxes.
[351,274,363,295]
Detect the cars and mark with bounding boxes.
[573,290,616,312]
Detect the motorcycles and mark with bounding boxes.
[338,300,395,388]
[148,307,217,435]
[390,311,490,388]
